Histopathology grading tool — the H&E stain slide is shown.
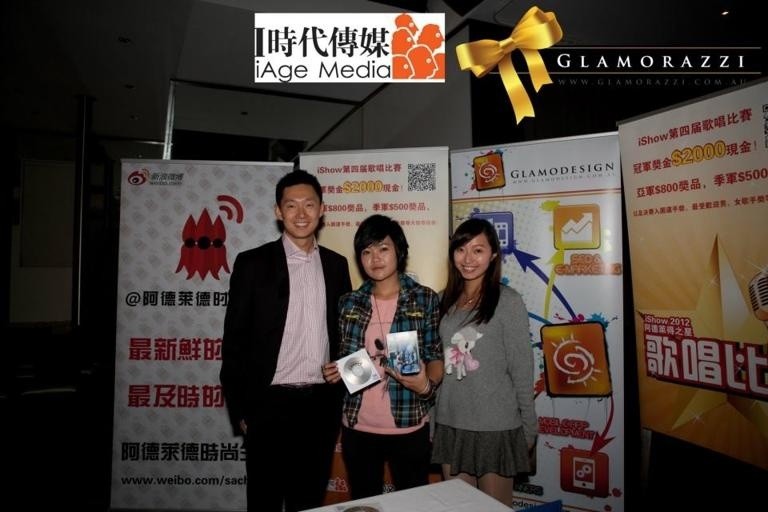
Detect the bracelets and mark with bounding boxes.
[418,377,432,394]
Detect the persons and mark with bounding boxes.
[321,215,447,501]
[429,217,539,509]
[219,170,354,511]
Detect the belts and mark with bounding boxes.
[272,384,321,398]
